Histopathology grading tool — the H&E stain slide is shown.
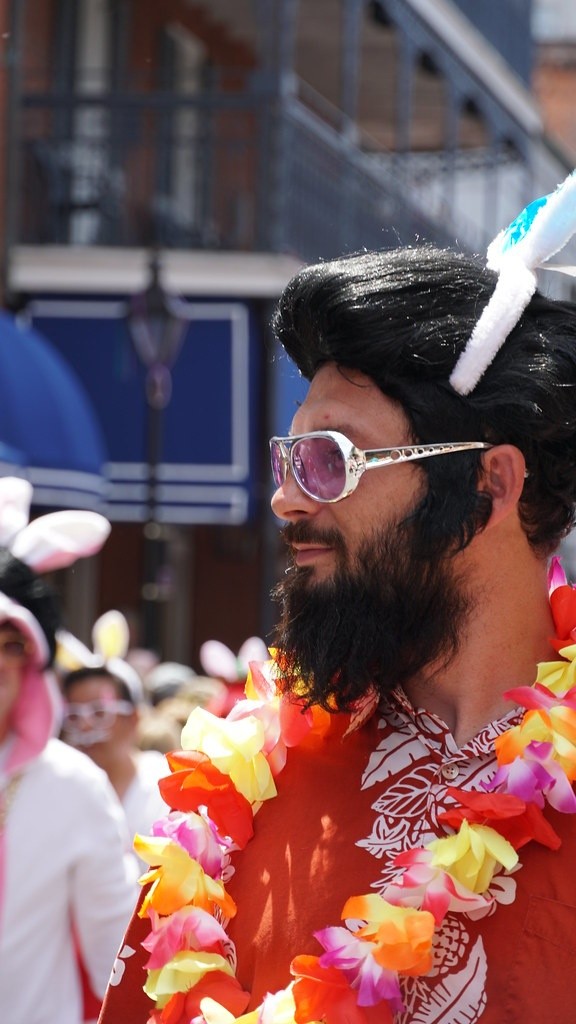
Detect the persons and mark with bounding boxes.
[59,668,172,878]
[0,592,142,1024]
[98,246,576,1024]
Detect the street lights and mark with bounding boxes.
[124,256,186,653]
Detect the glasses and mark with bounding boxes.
[269,425,531,505]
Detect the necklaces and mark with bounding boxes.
[132,552,576,1024]
